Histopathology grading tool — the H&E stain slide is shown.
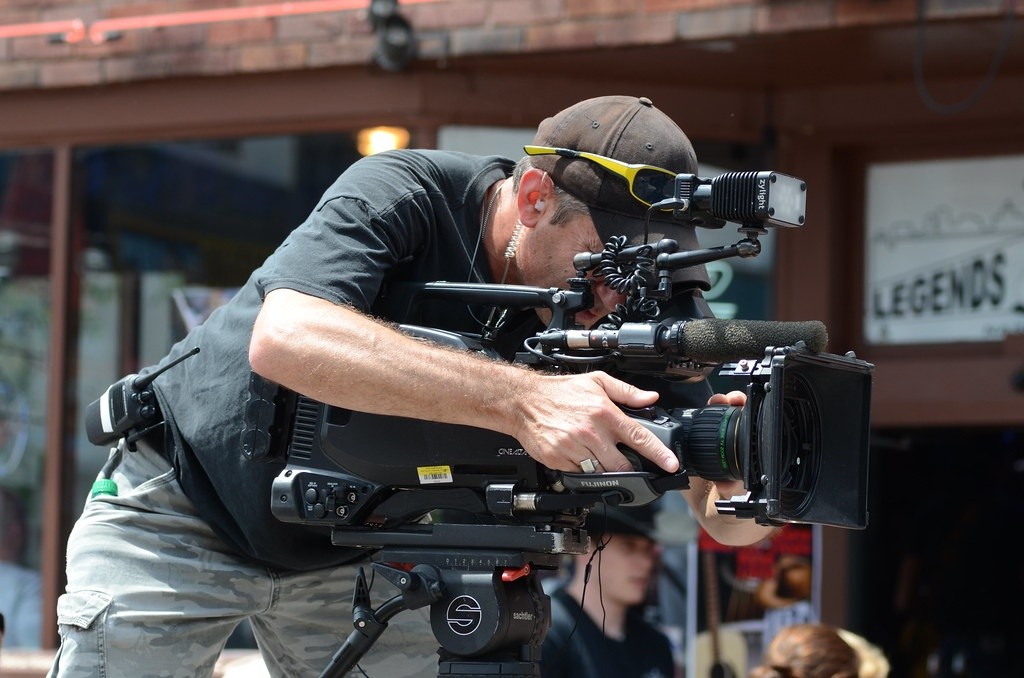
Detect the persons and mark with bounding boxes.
[748,619,890,678]
[532,499,676,678]
[40,98,775,678]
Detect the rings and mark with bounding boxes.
[580,458,599,473]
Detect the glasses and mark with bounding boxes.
[523,145,678,213]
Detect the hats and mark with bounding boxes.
[588,495,664,542]
[527,96,711,292]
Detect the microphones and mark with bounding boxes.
[662,320,827,361]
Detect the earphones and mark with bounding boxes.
[535,199,545,211]
